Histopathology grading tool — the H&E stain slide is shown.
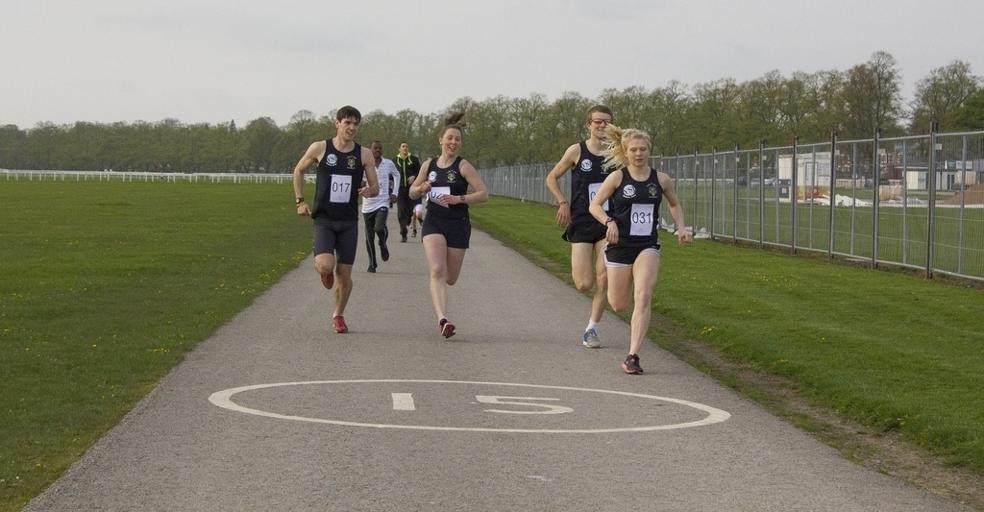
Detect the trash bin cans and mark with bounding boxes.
[879,178,889,201]
[778,179,791,198]
[888,179,903,200]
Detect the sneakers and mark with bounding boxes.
[332,313,348,333]
[380,246,389,261]
[583,326,602,348]
[367,263,378,273]
[320,272,335,290]
[437,316,457,339]
[399,218,424,242]
[622,353,643,374]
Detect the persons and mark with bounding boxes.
[361,139,400,273]
[409,194,423,237]
[544,104,623,349]
[587,122,694,375]
[392,142,421,243]
[292,104,380,335]
[407,110,488,341]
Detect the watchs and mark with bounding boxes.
[604,217,614,227]
[294,197,303,204]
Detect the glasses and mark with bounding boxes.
[591,117,613,124]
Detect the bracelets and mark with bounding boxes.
[559,200,568,205]
[459,194,466,204]
